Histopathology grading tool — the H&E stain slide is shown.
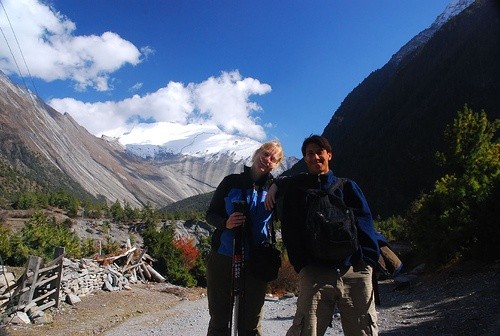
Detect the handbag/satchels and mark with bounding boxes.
[259,244,282,281]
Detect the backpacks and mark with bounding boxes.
[295,176,360,261]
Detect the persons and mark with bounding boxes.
[205,141,283,335]
[280,132,381,336]
